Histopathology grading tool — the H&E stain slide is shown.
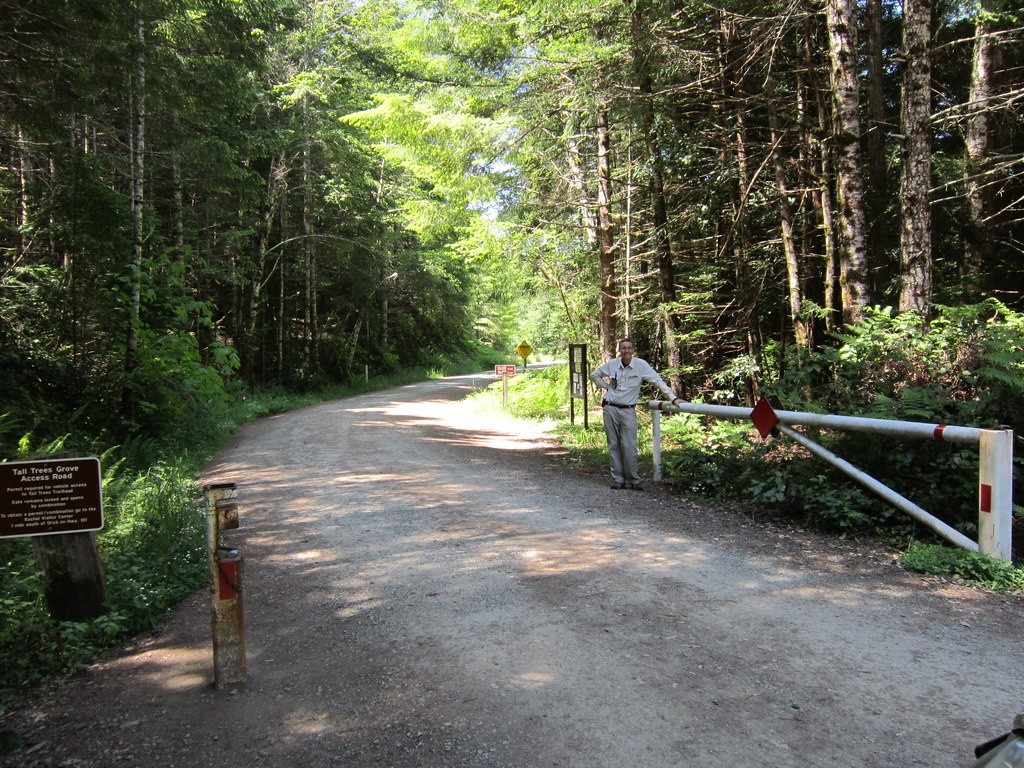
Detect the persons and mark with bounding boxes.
[590,338,689,492]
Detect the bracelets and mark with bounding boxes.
[672,398,677,404]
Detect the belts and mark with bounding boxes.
[605,401,636,409]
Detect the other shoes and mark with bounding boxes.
[611,481,625,489]
[631,483,644,491]
